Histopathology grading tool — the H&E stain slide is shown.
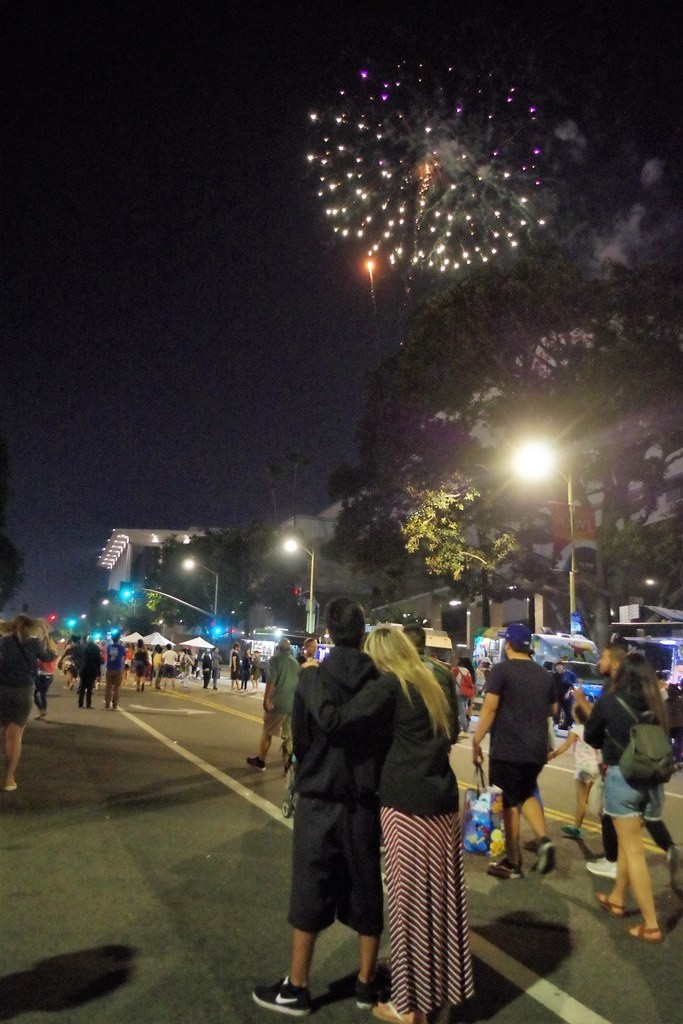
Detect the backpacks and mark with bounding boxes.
[604,693,676,785]
[455,667,475,697]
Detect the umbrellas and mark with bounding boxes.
[143,631,176,650]
[179,636,215,654]
[120,631,143,643]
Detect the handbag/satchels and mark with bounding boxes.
[462,761,506,858]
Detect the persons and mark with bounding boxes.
[0,614,57,792]
[474,622,561,879]
[298,625,476,1024]
[202,651,212,689]
[231,642,261,694]
[133,639,177,690]
[211,647,222,690]
[246,637,321,771]
[570,643,683,942]
[176,647,203,680]
[402,626,461,733]
[251,597,392,1015]
[428,652,492,731]
[62,632,135,710]
[526,662,603,851]
[33,658,56,719]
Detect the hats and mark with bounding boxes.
[498,623,532,647]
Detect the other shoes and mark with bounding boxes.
[4,779,18,792]
[532,836,556,875]
[87,706,94,709]
[119,682,260,692]
[486,859,523,880]
[559,822,584,840]
[34,712,47,720]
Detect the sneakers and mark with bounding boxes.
[252,976,311,1016]
[354,968,380,1010]
[586,856,619,880]
[665,844,683,893]
[246,756,266,771]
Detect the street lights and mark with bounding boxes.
[102,599,116,628]
[448,599,472,659]
[509,438,579,635]
[283,540,316,636]
[182,559,220,648]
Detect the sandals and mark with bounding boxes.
[595,890,627,917]
[628,922,665,944]
[373,999,428,1024]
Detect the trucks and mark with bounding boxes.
[362,622,455,664]
[470,625,604,731]
[623,635,683,764]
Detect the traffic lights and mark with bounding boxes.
[231,628,236,636]
[119,582,134,601]
